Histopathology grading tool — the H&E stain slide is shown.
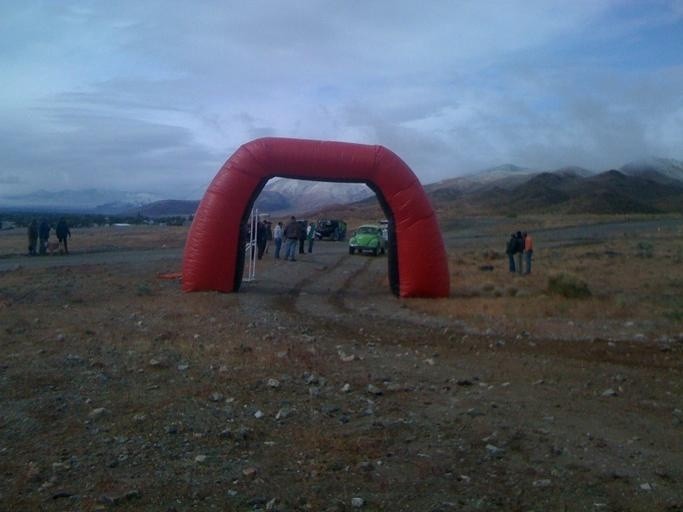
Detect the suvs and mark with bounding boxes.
[316,219,346,241]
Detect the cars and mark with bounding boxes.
[348,224,385,256]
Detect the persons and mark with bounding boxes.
[307,223,317,253]
[283,216,298,261]
[265,222,273,254]
[505,233,517,273]
[273,222,283,258]
[298,220,308,254]
[38,220,50,255]
[55,216,71,255]
[27,218,38,256]
[252,215,262,260]
[522,231,533,274]
[261,220,266,257]
[516,231,524,274]
